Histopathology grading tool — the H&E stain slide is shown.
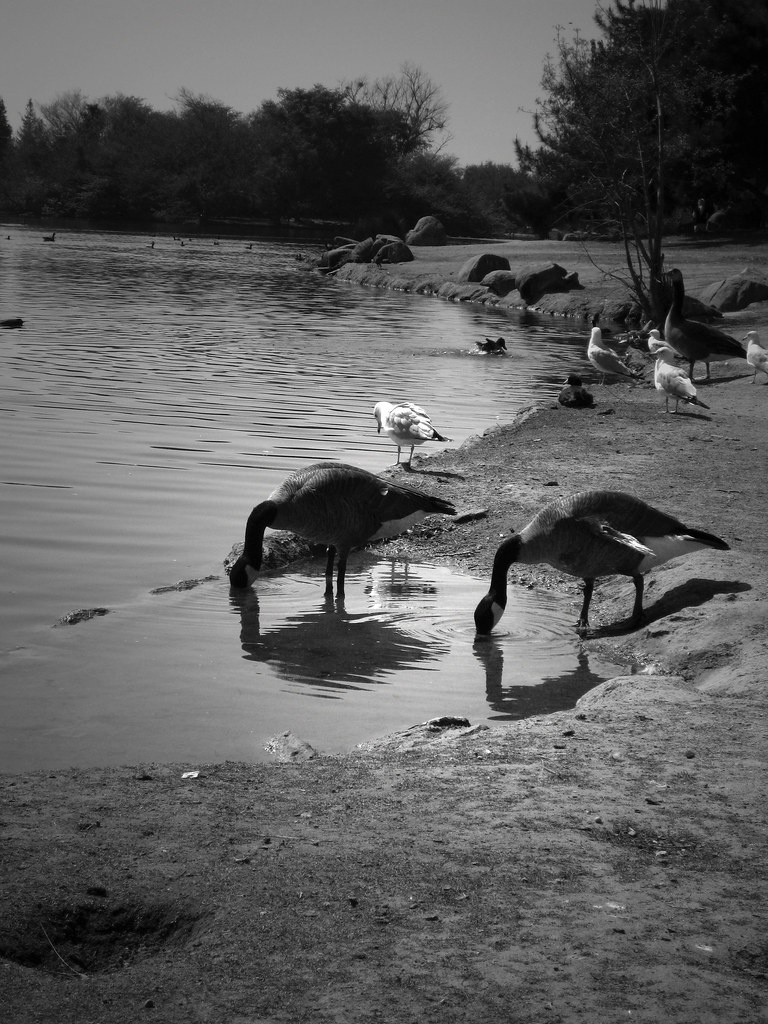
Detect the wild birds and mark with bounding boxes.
[245,244,252,249]
[742,330,768,385]
[180,242,184,246]
[648,346,710,413]
[587,327,645,386]
[647,329,684,360]
[475,336,508,356]
[373,402,445,464]
[189,238,192,242]
[473,490,731,637]
[42,232,56,241]
[5,235,11,239]
[663,268,748,385]
[213,241,219,245]
[173,236,181,241]
[228,461,457,599]
[146,240,154,248]
[559,375,593,407]
